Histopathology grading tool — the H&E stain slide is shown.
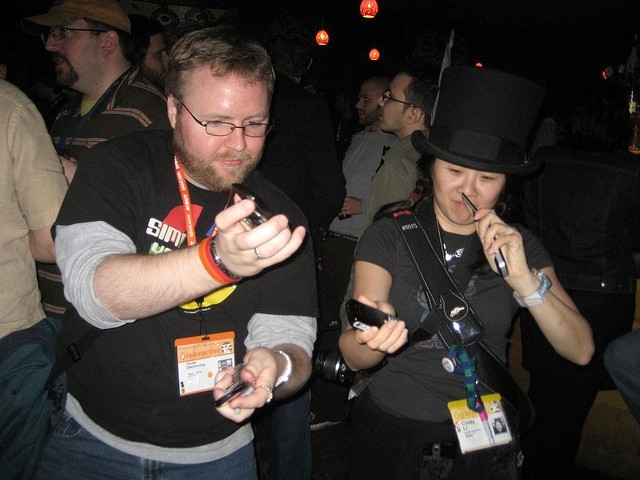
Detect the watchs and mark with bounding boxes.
[506,269,554,305]
[275,345,295,394]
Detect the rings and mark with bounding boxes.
[255,248,261,259]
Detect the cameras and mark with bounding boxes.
[314,345,356,386]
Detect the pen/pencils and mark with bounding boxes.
[209,379,253,409]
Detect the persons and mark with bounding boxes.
[322,70,393,326]
[337,63,594,478]
[36,29,317,479]
[35,2,167,338]
[0,59,68,478]
[524,76,636,480]
[345,73,437,326]
[119,13,172,80]
[263,25,345,233]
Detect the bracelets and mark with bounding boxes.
[200,234,247,287]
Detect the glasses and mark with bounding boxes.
[381,88,422,109]
[40,25,109,46]
[172,94,275,138]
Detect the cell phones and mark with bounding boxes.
[208,362,247,407]
[345,298,415,341]
[231,183,273,226]
[460,192,509,279]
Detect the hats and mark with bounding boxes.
[410,65,553,178]
[29,0,133,34]
